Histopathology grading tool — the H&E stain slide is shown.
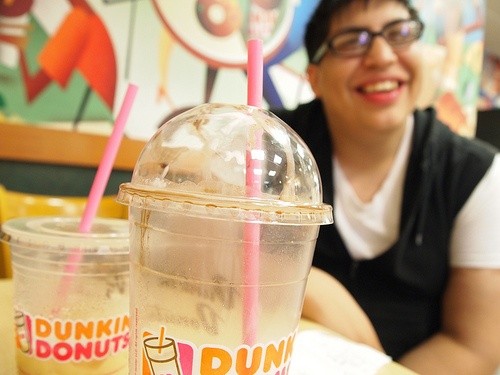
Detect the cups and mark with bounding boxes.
[0,215,129,374]
[115,104,333,374]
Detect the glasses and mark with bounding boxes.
[312,18,424,64]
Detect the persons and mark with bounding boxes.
[266,0,500,375]
